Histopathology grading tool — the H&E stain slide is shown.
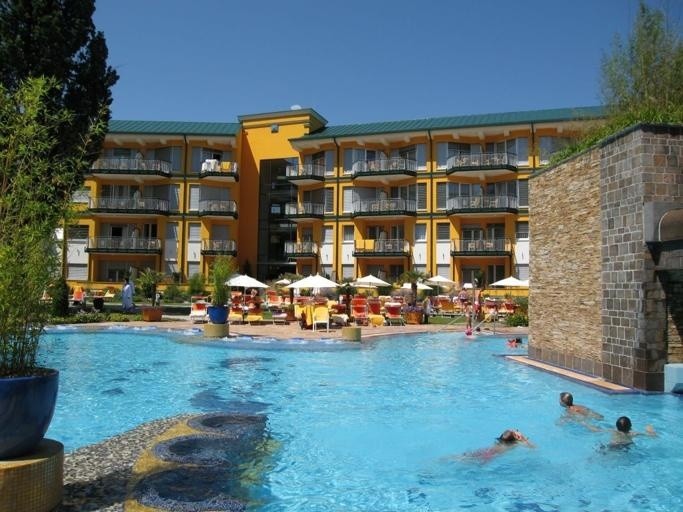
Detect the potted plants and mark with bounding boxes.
[0,74,110,461]
[201,245,243,325]
[397,266,433,325]
[136,267,171,321]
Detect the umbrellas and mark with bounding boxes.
[339,277,377,294]
[283,273,343,297]
[399,280,433,301]
[294,270,342,294]
[488,276,529,302]
[222,274,271,315]
[351,274,391,296]
[424,275,457,296]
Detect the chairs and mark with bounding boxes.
[40,286,117,305]
[190,290,522,334]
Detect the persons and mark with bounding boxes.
[464,324,473,336]
[462,301,473,328]
[483,304,498,323]
[505,336,523,350]
[457,287,468,313]
[431,428,538,467]
[119,277,135,314]
[593,414,660,458]
[475,327,481,334]
[472,286,484,320]
[554,391,608,433]
[420,294,431,324]
